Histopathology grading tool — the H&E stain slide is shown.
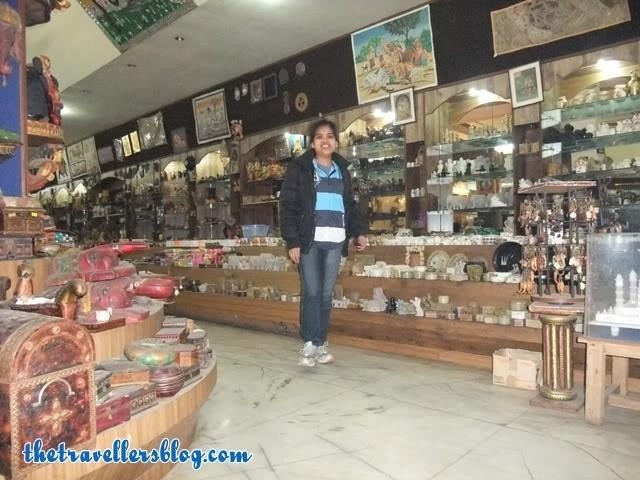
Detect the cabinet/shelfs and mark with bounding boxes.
[0,39,638,395]
[0,190,212,480]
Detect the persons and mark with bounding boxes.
[281,120,371,366]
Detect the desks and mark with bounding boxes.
[576,335,640,424]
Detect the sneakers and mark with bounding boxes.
[299,341,318,368]
[316,342,335,364]
